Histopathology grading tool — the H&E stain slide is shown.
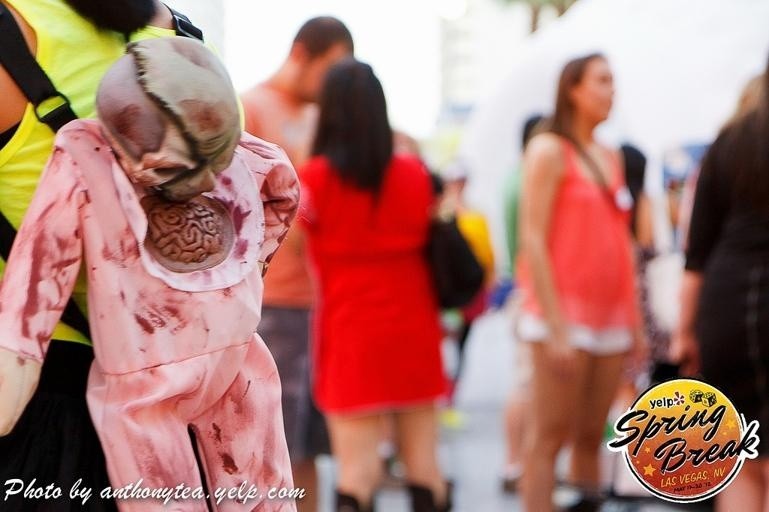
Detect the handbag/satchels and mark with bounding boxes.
[424,216,482,310]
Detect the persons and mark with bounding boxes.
[293,57,456,512]
[665,50,769,511]
[231,17,362,511]
[510,51,647,511]
[0,1,209,512]
[670,69,769,263]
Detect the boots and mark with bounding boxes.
[334,481,455,511]
